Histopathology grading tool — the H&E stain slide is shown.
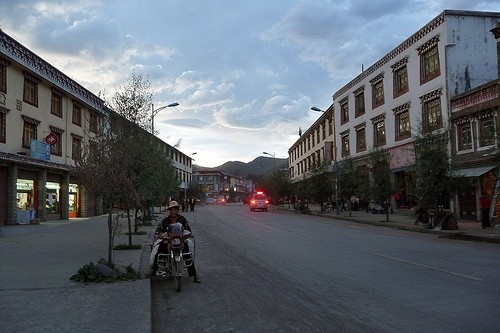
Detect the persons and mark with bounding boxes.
[180,195,196,212]
[150,201,202,283]
[394,188,406,208]
[17,198,21,209]
[331,191,369,213]
[46,199,59,210]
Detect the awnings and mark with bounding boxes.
[447,166,497,178]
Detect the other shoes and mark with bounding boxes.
[149,273,155,277]
[195,279,202,283]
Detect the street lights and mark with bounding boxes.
[263,151,275,173]
[310,105,341,215]
[150,102,180,135]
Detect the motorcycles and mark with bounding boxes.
[154,222,194,293]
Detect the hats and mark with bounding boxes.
[166,201,180,209]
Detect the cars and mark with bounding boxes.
[217,197,226,206]
[206,198,216,205]
[250,193,267,212]
